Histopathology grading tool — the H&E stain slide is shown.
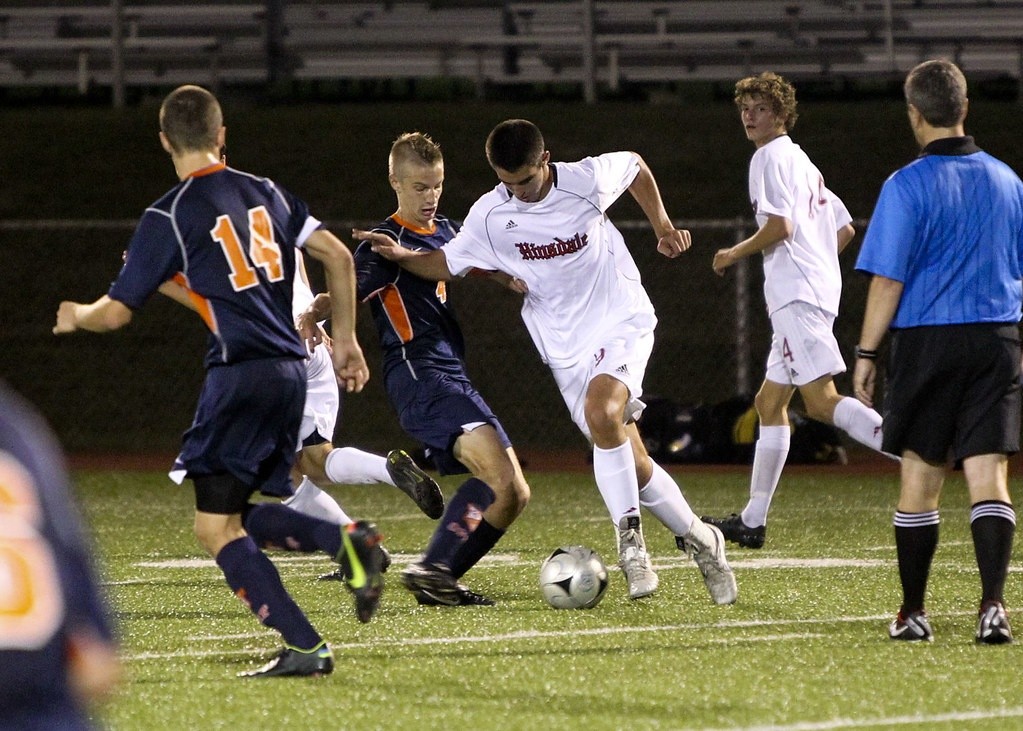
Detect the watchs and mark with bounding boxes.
[853,345,878,359]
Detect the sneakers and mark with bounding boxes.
[889,605,934,643]
[701,513,767,549]
[330,520,387,622]
[975,600,1013,645]
[612,514,659,599]
[386,449,445,519]
[235,640,334,677]
[317,546,391,582]
[400,562,496,606]
[675,522,737,604]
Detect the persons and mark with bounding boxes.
[53,84,738,682]
[700,71,902,549]
[0,381,122,731]
[852,58,1023,645]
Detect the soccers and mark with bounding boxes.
[538,545,611,610]
